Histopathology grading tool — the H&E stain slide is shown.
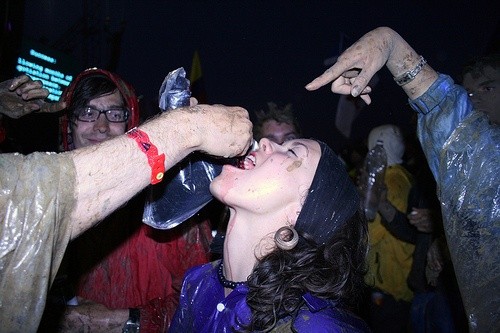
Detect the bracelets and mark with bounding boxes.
[125,126,165,184]
[394,55,427,86]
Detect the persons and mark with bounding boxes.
[0,66,214,333]
[167,139,370,333]
[305,27,500,333]
[255,103,469,332]
[454,58,499,130]
[0,104,254,333]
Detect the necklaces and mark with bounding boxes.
[218,259,248,289]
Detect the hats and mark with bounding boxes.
[368,125,404,167]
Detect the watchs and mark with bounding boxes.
[122,308,142,333]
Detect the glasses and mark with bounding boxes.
[73,106,130,123]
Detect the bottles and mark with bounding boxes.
[356,139,387,222]
